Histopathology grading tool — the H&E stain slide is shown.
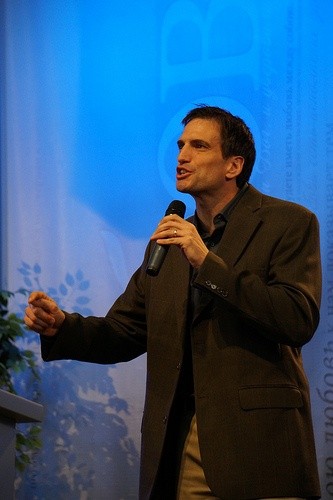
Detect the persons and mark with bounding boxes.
[21,102,323,500]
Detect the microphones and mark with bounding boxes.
[145,199,186,277]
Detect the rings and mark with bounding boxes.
[174,230,177,236]
